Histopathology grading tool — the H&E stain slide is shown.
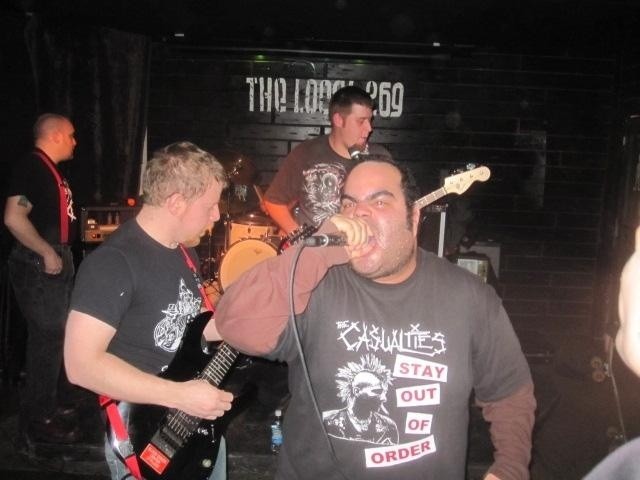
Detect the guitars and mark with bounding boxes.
[279,160,491,255]
[106,310,261,479]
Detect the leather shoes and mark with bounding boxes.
[22,405,81,444]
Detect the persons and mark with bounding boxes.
[212,159,539,479]
[582,226,640,480]
[60,140,236,479]
[257,87,376,241]
[4,112,83,443]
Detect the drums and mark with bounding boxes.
[203,280,223,309]
[228,215,278,244]
[217,237,277,292]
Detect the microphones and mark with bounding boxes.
[348,145,360,159]
[306,234,347,247]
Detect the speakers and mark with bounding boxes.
[459,239,502,282]
